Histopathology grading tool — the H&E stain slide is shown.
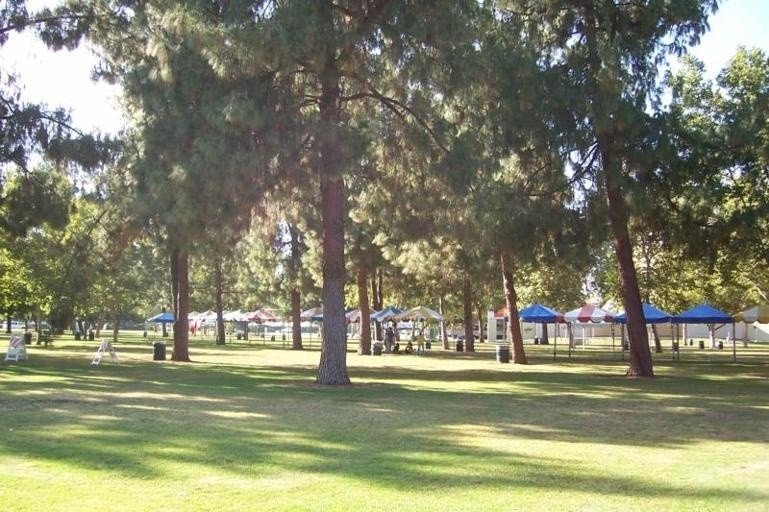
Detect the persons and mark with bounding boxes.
[405,342,412,353]
[190,325,194,334]
[417,332,426,355]
[393,343,399,352]
[373,326,393,355]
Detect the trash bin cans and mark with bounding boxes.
[23,332,31,344]
[153,341,166,360]
[89,331,95,341]
[75,331,81,340]
[496,345,509,363]
[455,340,463,352]
